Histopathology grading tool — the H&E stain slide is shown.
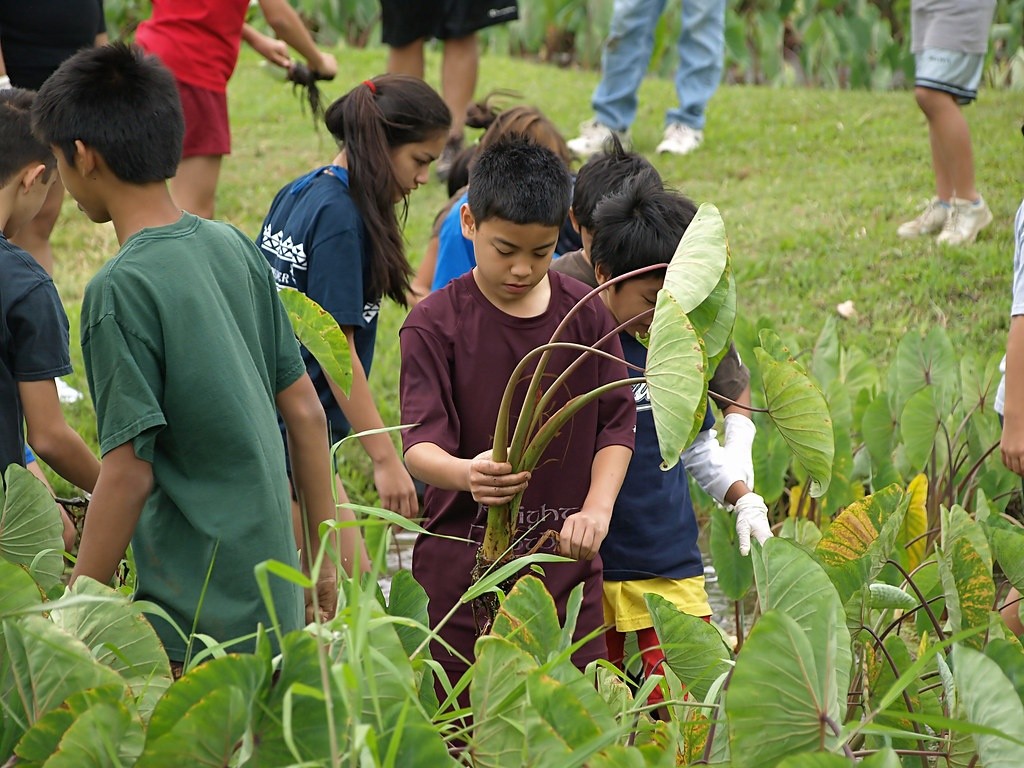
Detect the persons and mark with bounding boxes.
[29,41,340,683]
[135,0,338,220]
[378,0,520,181]
[399,131,636,768]
[566,0,725,160]
[896,0,997,246]
[405,87,579,308]
[255,72,452,584]
[589,188,773,725]
[993,198,1024,640]
[0,0,108,281]
[550,128,756,513]
[0,86,106,555]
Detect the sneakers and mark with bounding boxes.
[655,122,703,154]
[936,190,993,248]
[566,119,631,160]
[897,196,950,238]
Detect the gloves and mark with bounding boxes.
[734,492,774,556]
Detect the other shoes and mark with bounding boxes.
[54,376,83,404]
[435,137,466,182]
